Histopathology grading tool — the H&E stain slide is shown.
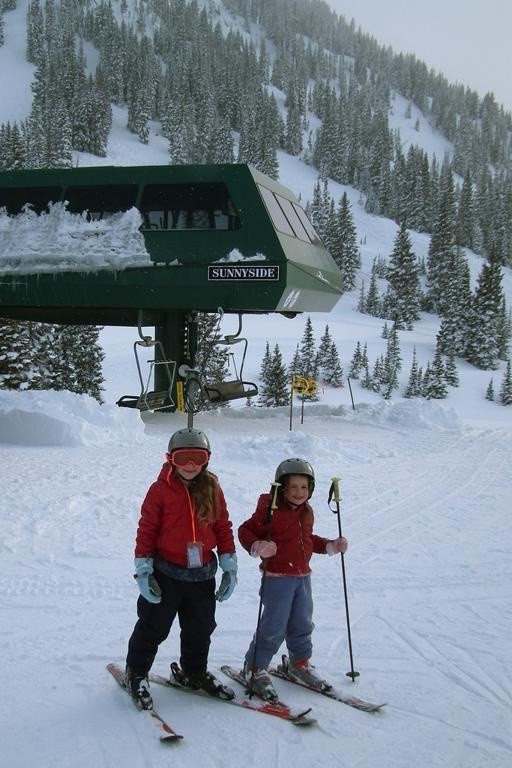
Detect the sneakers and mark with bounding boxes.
[183,658,326,703]
[127,667,153,710]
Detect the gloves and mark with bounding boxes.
[215,553,238,601]
[326,537,348,555]
[134,558,162,604]
[254,541,277,558]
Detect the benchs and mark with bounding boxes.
[197,338,258,403]
[118,340,178,413]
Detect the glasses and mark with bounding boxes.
[172,449,209,466]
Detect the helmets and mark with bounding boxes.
[276,458,315,489]
[168,428,211,454]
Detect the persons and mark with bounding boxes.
[240,456,348,707]
[122,427,239,712]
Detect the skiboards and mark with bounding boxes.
[221,656,388,726]
[106,662,312,743]
